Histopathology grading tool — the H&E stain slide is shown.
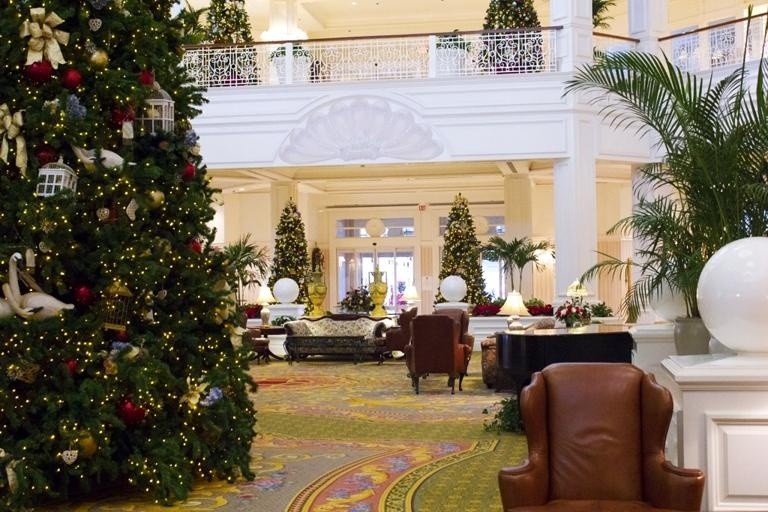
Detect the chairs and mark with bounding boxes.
[386,306,418,352]
[248,328,269,361]
[404,310,464,392]
[429,309,471,378]
[496,357,702,511]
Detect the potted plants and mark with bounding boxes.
[547,45,768,358]
[429,31,473,77]
[273,45,312,85]
[244,303,259,318]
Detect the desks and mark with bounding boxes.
[260,324,287,337]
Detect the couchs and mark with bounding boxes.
[479,318,554,390]
[284,314,389,365]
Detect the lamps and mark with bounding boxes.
[398,286,422,312]
[256,287,276,327]
[495,290,533,330]
[567,279,586,299]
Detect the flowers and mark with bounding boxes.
[556,301,590,328]
[469,299,552,315]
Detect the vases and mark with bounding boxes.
[368,269,389,316]
